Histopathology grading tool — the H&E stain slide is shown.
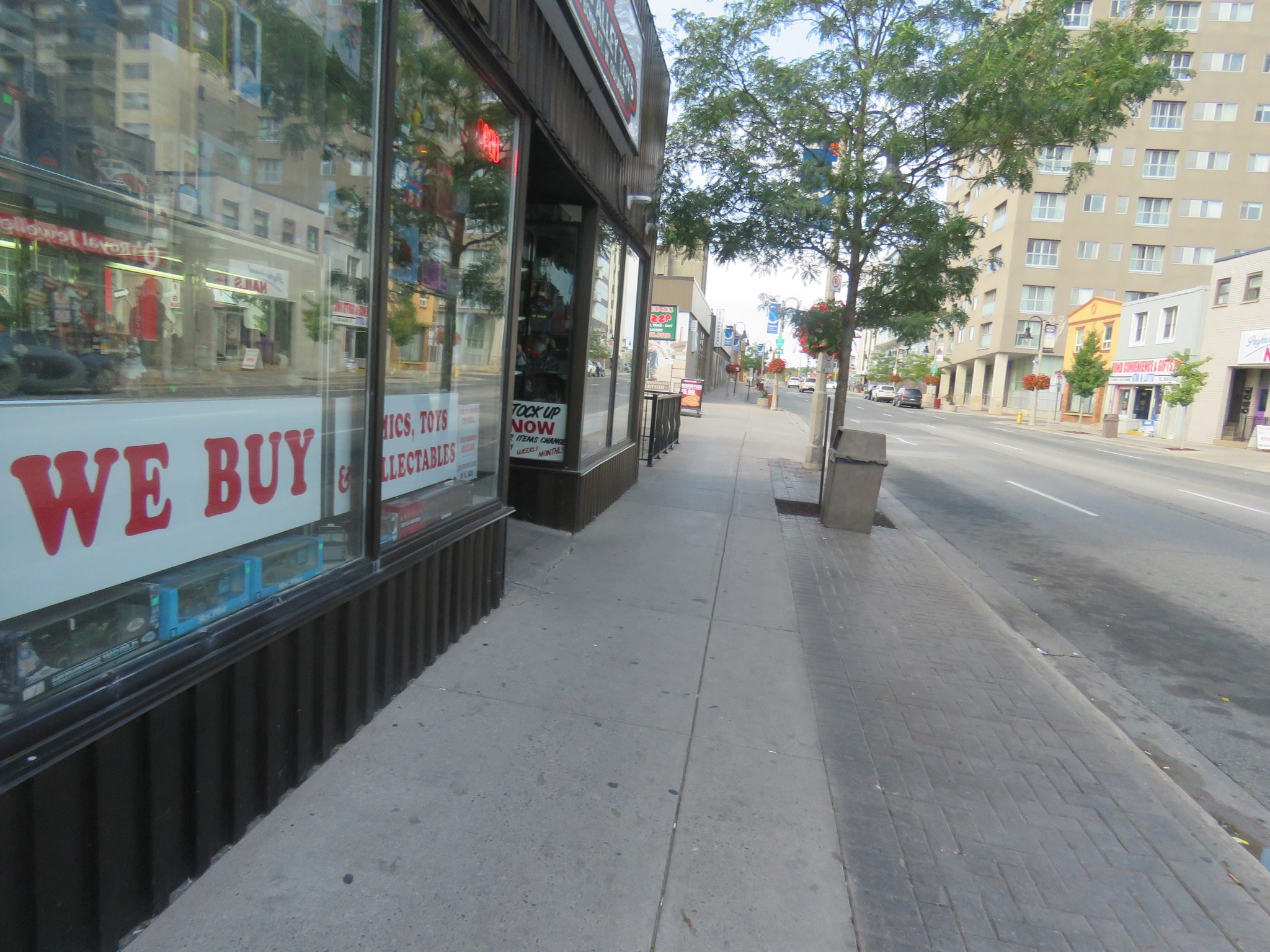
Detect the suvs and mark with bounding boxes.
[587,360,598,376]
[787,377,800,388]
[799,377,816,393]
[593,362,606,376]
[871,384,896,403]
[892,386,923,409]
[863,384,877,400]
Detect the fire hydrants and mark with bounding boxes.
[1017,411,1023,424]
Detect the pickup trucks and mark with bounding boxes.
[624,363,631,373]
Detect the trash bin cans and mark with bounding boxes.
[1102,414,1119,438]
[819,426,889,534]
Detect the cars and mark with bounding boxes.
[826,381,838,389]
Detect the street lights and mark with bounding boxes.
[889,345,900,390]
[923,337,937,386]
[770,297,801,411]
[864,349,873,361]
[792,354,801,371]
[725,321,749,399]
[760,341,774,381]
[1018,315,1049,427]
